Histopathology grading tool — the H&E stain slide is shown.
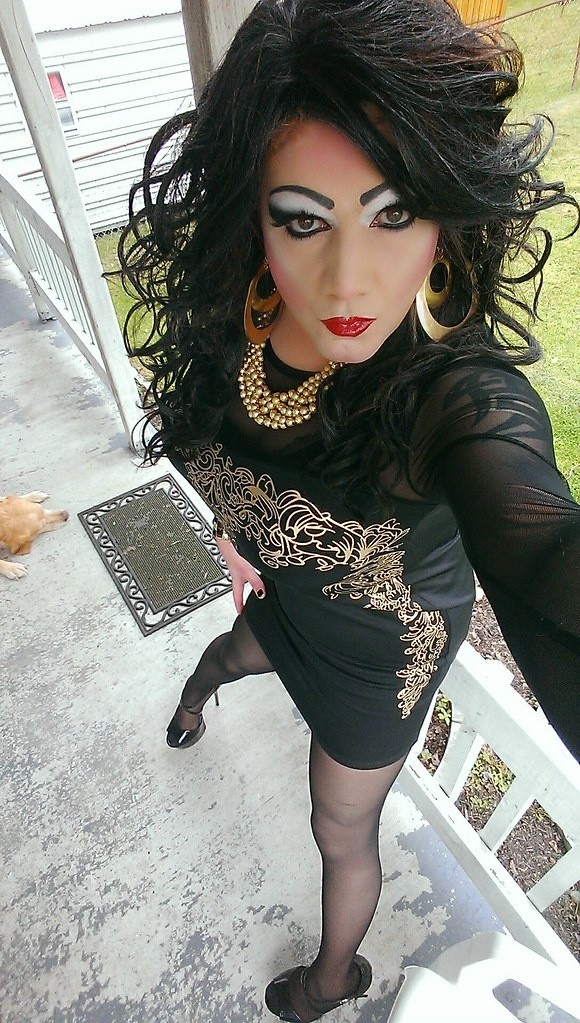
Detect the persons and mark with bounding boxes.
[100,0,580,1023]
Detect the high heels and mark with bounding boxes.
[265,954,372,1023]
[166,675,220,749]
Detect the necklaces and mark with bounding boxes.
[236,306,348,427]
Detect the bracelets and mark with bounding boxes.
[212,518,230,543]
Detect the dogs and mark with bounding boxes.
[0,489,69,580]
